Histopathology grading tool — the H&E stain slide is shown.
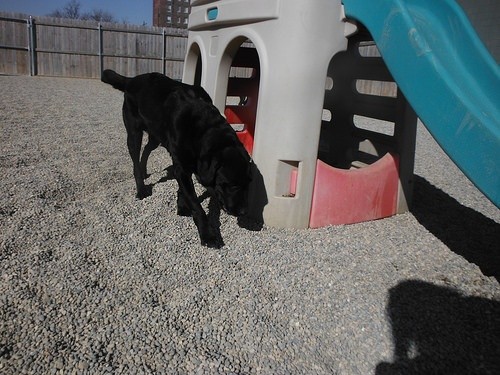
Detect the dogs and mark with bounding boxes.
[101,68,268,250]
